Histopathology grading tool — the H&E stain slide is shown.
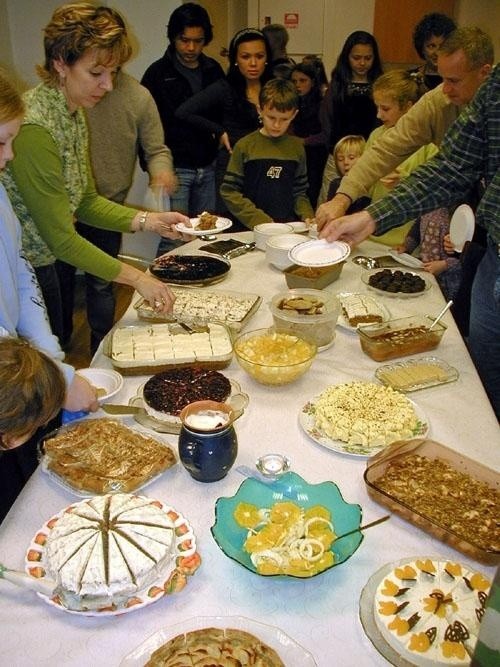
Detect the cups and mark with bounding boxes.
[176,401,238,483]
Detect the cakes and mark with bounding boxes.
[368,269,425,293]
[110,322,233,361]
[315,382,417,448]
[340,296,383,326]
[142,365,231,428]
[144,627,288,667]
[150,254,229,284]
[193,212,219,231]
[141,287,260,322]
[373,557,491,667]
[381,363,446,388]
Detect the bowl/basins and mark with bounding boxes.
[233,326,318,387]
[268,286,342,348]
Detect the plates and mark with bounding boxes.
[174,216,233,236]
[298,379,430,461]
[375,356,460,392]
[209,470,366,582]
[363,437,499,570]
[357,556,499,666]
[147,252,231,286]
[73,365,124,403]
[316,332,338,352]
[448,203,477,253]
[127,375,250,435]
[333,290,391,332]
[253,220,310,271]
[360,268,433,299]
[116,615,323,666]
[387,246,426,270]
[287,237,351,268]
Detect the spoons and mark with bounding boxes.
[197,234,218,241]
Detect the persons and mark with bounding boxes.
[222,11,496,313]
[0,81,98,470]
[135,3,235,220]
[3,337,65,449]
[320,61,499,411]
[1,3,193,355]
[62,63,177,352]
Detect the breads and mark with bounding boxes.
[42,417,177,493]
[278,294,326,315]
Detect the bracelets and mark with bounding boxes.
[139,211,148,231]
